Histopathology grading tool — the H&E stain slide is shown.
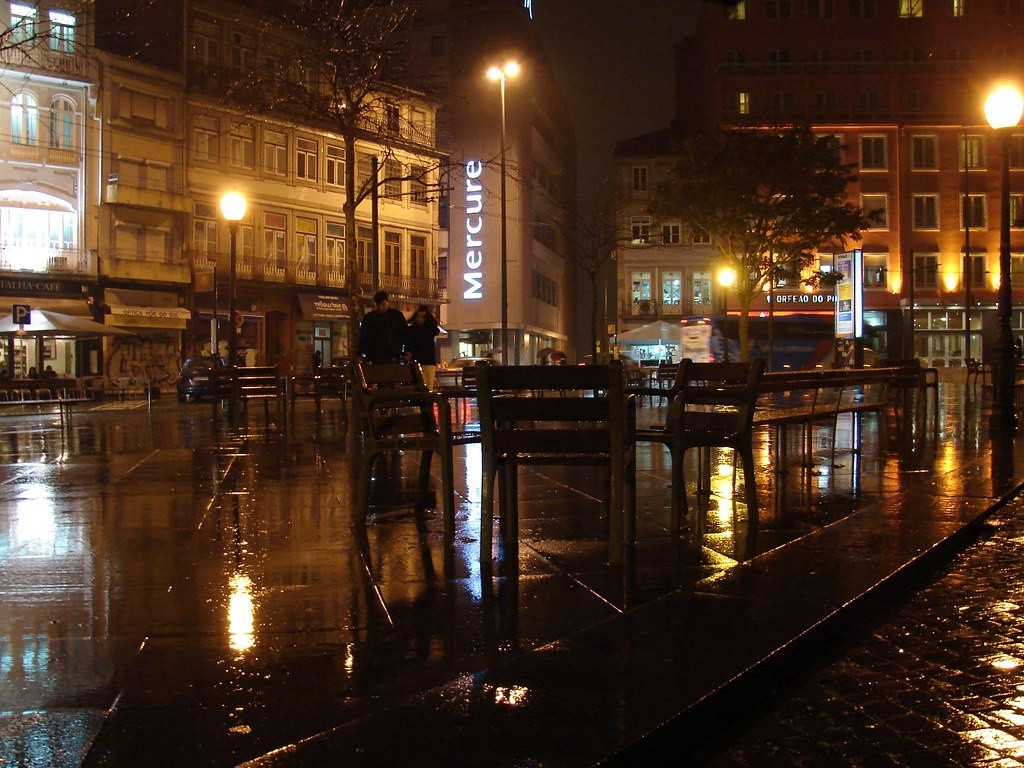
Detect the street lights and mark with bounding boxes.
[487,60,521,367]
[718,266,736,362]
[219,186,247,408]
[985,82,1024,479]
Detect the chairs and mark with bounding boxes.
[474,361,636,569]
[623,358,768,532]
[346,358,483,535]
[965,357,992,388]
[291,363,349,419]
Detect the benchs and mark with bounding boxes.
[433,366,479,391]
[656,364,679,390]
[687,358,942,536]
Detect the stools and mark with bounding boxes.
[0,385,81,403]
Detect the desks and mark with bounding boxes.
[12,378,78,388]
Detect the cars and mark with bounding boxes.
[176,355,234,403]
[447,358,500,389]
[319,356,366,393]
[578,355,594,367]
[609,354,645,386]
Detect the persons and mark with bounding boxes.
[24,366,40,379]
[212,342,259,387]
[403,304,440,414]
[313,350,322,377]
[1013,338,1022,385]
[558,358,567,366]
[43,365,58,378]
[539,356,551,367]
[355,290,412,419]
[0,369,12,383]
[484,354,494,367]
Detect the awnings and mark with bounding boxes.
[104,301,191,329]
[679,311,835,324]
[0,296,95,321]
[193,307,265,322]
[295,292,355,321]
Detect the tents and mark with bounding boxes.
[609,318,680,386]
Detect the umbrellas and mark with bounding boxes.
[535,347,555,358]
[225,342,250,349]
[484,349,502,354]
[0,307,138,377]
[550,350,567,360]
